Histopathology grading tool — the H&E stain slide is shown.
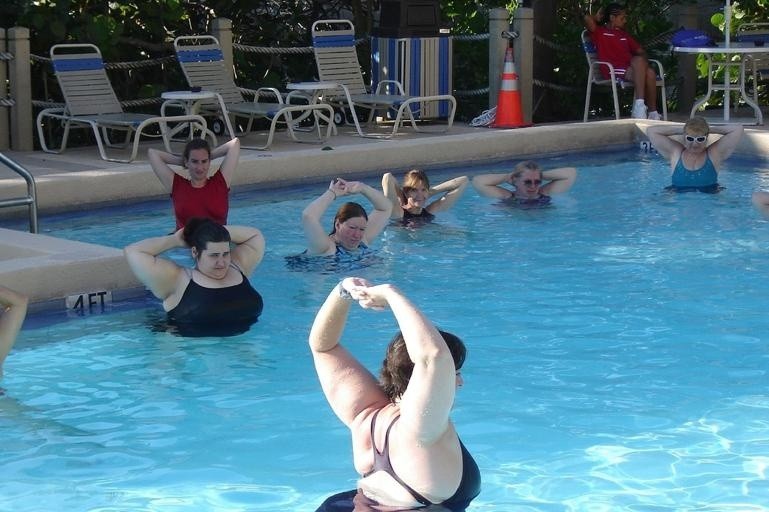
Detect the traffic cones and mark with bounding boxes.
[486,43,536,132]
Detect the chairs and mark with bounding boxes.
[737,23,769,118]
[174,36,335,149]
[582,29,668,121]
[36,44,207,163]
[312,20,457,139]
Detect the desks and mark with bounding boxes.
[670,42,769,126]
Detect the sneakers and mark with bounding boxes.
[630,104,648,120]
[648,111,662,121]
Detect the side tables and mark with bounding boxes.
[287,82,338,139]
[160,91,215,157]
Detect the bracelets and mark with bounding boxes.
[328,189,337,200]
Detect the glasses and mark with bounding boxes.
[519,179,542,187]
[684,135,708,144]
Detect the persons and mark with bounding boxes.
[751,191,769,219]
[146,136,240,236]
[646,116,744,192]
[472,160,577,209]
[124,218,265,336]
[285,178,393,274]
[0,284,86,436]
[584,3,661,120]
[309,278,481,512]
[382,169,469,241]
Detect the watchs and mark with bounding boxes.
[339,281,352,300]
